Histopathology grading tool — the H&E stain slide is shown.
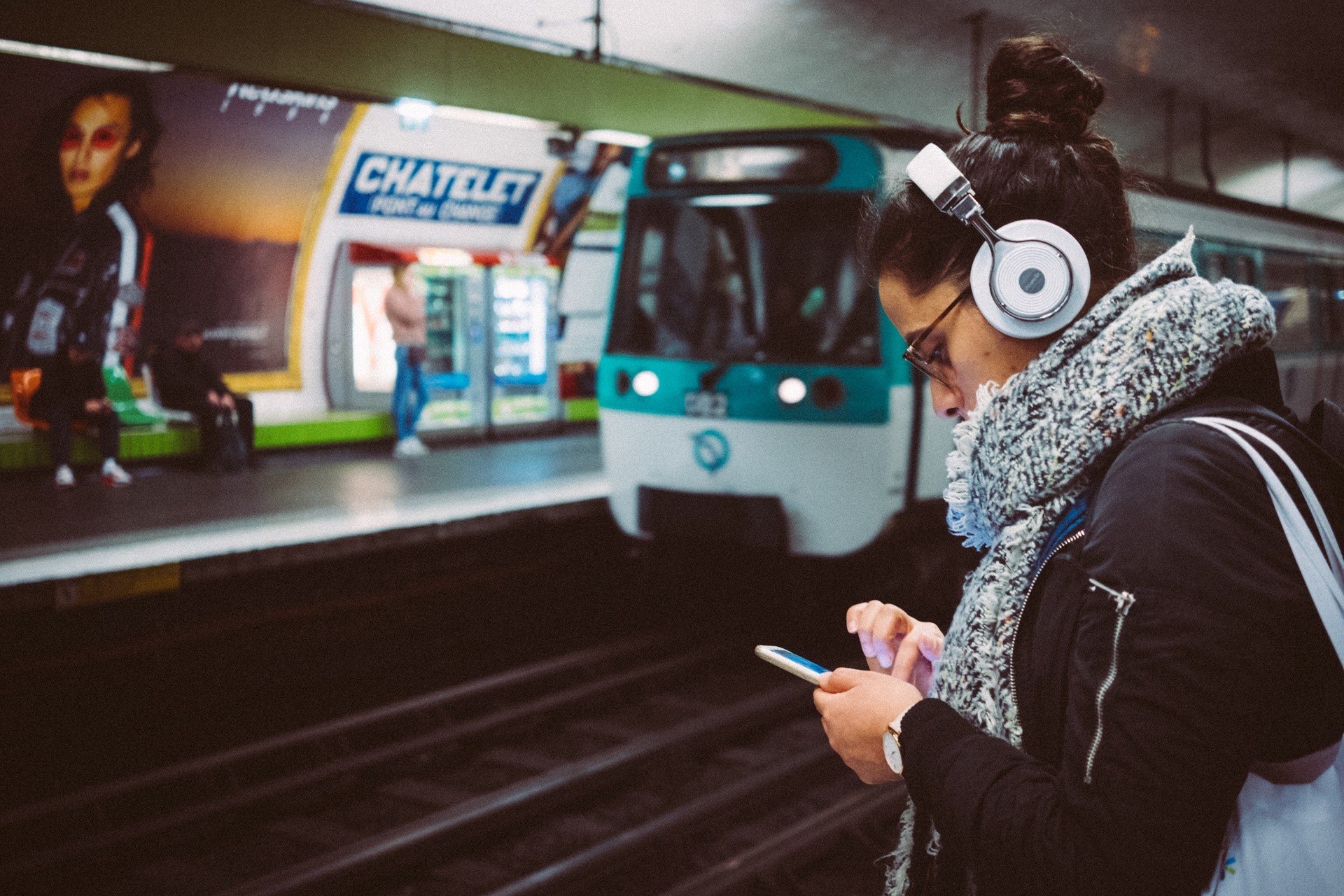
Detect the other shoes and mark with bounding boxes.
[391,436,429,460]
[100,464,131,488]
[52,464,74,490]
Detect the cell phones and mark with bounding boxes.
[755,645,834,684]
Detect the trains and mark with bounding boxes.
[596,123,1343,599]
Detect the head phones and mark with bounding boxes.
[905,143,1091,340]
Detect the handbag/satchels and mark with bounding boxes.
[1181,412,1343,896]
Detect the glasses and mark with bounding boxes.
[60,123,128,153]
[902,284,972,387]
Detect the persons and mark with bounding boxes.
[385,247,431,461]
[813,31,1344,896]
[28,331,134,498]
[0,68,163,382]
[159,320,254,477]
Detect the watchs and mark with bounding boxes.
[882,708,918,788]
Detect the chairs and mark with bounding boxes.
[10,366,91,432]
[102,364,162,427]
[141,365,198,425]
